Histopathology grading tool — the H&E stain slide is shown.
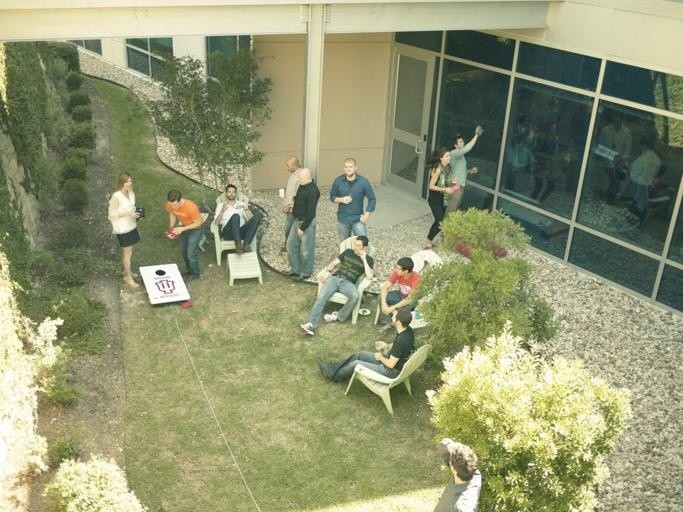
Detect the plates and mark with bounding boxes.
[358,308,371,315]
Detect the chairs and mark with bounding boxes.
[618,174,670,229]
[373,249,443,331]
[209,187,261,267]
[315,235,374,324]
[345,343,434,417]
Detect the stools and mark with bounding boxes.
[226,252,264,285]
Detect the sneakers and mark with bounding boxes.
[297,322,316,337]
[323,310,338,323]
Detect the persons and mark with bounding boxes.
[427,149,458,248]
[507,138,537,191]
[528,123,560,201]
[282,159,302,251]
[597,111,633,203]
[650,164,675,218]
[571,104,594,165]
[433,438,481,512]
[620,138,661,227]
[285,169,320,281]
[107,173,141,289]
[165,191,203,282]
[331,159,375,239]
[317,310,416,384]
[301,236,374,335]
[517,116,537,147]
[445,128,483,213]
[379,258,424,332]
[216,184,263,253]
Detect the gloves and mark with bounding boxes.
[473,124,484,135]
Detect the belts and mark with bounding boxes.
[294,216,305,222]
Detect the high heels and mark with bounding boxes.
[122,271,138,279]
[423,242,435,250]
[122,278,141,290]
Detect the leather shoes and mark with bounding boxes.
[280,271,296,277]
[292,274,311,282]
[185,274,201,282]
[234,239,244,256]
[242,240,252,253]
[315,356,330,380]
[380,323,396,335]
[181,270,191,277]
